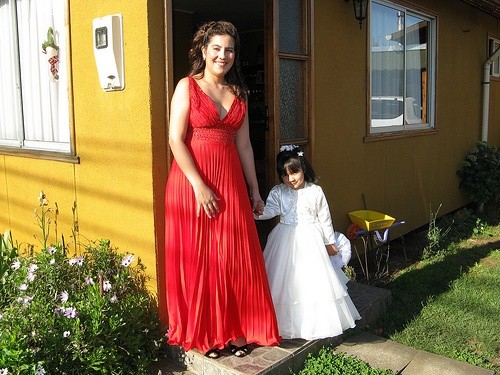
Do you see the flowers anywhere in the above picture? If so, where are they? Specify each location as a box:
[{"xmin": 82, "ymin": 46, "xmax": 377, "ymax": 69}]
[{"xmin": 297, "ymin": 151, "xmax": 304, "ymax": 157}]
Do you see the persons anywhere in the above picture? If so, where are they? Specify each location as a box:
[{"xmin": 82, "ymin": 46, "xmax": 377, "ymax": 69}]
[
  {"xmin": 163, "ymin": 21, "xmax": 282, "ymax": 357},
  {"xmin": 253, "ymin": 144, "xmax": 361, "ymax": 340}
]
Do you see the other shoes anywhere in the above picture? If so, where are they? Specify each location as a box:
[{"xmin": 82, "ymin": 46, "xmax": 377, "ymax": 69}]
[
  {"xmin": 205, "ymin": 349, "xmax": 221, "ymax": 359},
  {"xmin": 230, "ymin": 344, "xmax": 247, "ymax": 357}
]
